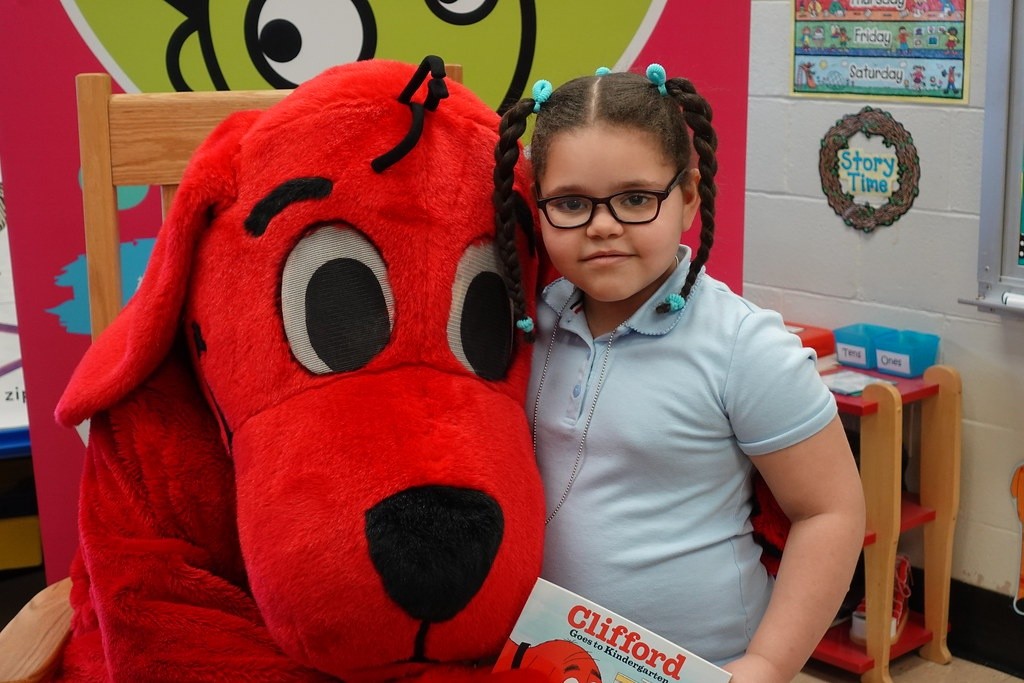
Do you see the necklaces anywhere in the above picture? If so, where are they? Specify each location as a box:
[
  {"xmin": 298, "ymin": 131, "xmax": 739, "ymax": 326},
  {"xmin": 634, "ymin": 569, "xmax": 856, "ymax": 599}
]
[{"xmin": 533, "ymin": 256, "xmax": 679, "ymax": 524}]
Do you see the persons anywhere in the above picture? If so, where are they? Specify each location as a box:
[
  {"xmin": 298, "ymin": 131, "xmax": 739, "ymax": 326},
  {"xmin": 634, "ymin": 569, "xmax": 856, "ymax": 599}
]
[{"xmin": 492, "ymin": 63, "xmax": 867, "ymax": 683}]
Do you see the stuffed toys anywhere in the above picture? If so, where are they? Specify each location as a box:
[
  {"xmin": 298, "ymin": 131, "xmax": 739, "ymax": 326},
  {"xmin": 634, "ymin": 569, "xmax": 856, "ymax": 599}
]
[{"xmin": 0, "ymin": 55, "xmax": 553, "ymax": 683}]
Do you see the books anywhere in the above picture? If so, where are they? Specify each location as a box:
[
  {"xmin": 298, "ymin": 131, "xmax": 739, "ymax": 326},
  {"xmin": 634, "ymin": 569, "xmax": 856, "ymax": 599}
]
[{"xmin": 493, "ymin": 577, "xmax": 732, "ymax": 683}]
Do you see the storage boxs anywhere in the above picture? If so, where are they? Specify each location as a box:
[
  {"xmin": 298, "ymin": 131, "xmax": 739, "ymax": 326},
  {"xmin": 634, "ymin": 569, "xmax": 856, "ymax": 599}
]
[
  {"xmin": 783, "ymin": 322, "xmax": 836, "ymax": 358},
  {"xmin": 873, "ymin": 330, "xmax": 941, "ymax": 379},
  {"xmin": 834, "ymin": 324, "xmax": 898, "ymax": 370}
]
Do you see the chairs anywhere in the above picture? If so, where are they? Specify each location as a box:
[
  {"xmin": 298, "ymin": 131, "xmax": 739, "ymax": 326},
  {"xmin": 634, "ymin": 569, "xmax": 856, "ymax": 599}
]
[{"xmin": 74, "ymin": 63, "xmax": 463, "ymax": 348}]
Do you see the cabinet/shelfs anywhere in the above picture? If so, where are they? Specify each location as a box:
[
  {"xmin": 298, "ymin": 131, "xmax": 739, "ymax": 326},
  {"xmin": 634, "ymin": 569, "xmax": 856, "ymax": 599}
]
[{"xmin": 747, "ymin": 353, "xmax": 962, "ymax": 683}]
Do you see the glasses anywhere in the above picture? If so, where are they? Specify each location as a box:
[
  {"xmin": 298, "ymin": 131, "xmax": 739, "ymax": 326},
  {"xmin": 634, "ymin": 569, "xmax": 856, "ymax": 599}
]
[{"xmin": 536, "ymin": 167, "xmax": 688, "ymax": 229}]
